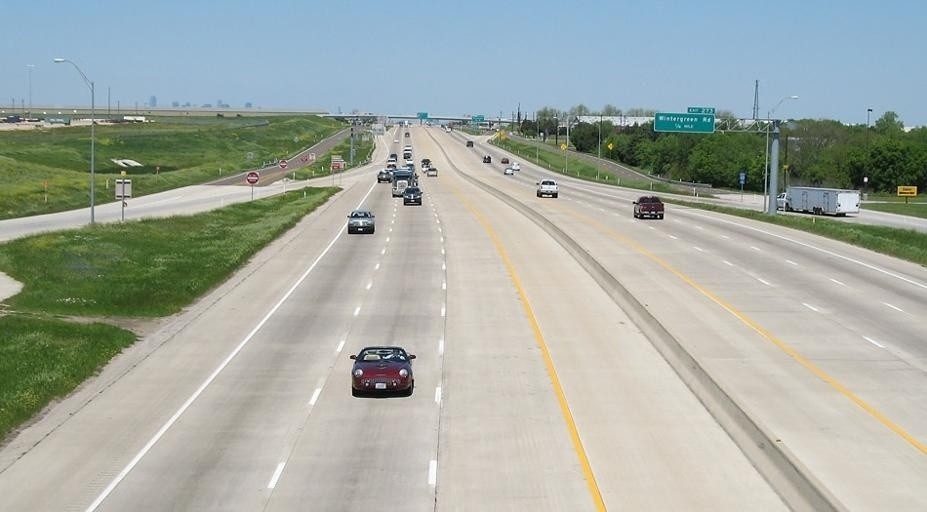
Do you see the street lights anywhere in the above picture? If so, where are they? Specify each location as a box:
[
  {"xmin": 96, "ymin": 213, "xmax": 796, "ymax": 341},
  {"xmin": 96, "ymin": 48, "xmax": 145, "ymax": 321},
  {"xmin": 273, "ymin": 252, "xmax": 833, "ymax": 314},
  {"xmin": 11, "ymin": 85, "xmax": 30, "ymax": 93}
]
[
  {"xmin": 554, "ymin": 117, "xmax": 580, "ymax": 175},
  {"xmin": 763, "ymin": 95, "xmax": 799, "ymax": 214},
  {"xmin": 54, "ymin": 58, "xmax": 93, "ymax": 224}
]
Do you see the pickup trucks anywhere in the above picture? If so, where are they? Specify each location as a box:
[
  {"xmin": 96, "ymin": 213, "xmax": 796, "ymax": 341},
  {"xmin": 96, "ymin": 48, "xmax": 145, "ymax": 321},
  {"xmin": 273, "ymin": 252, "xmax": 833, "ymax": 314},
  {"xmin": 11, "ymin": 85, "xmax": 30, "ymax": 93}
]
[
  {"xmin": 633, "ymin": 196, "xmax": 663, "ymax": 220},
  {"xmin": 536, "ymin": 179, "xmax": 557, "ymax": 198}
]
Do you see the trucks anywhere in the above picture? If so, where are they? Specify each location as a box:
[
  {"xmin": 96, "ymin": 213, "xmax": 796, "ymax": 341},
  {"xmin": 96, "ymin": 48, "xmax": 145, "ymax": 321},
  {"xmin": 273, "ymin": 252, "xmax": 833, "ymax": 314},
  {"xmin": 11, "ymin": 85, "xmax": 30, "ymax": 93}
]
[{"xmin": 778, "ymin": 185, "xmax": 862, "ymax": 216}]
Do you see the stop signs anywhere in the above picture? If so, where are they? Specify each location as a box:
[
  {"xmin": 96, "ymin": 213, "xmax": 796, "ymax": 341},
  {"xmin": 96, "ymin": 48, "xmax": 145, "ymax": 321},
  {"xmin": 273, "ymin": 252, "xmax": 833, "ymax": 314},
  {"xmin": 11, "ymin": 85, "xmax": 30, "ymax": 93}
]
[
  {"xmin": 246, "ymin": 171, "xmax": 259, "ymax": 185},
  {"xmin": 279, "ymin": 160, "xmax": 288, "ymax": 168}
]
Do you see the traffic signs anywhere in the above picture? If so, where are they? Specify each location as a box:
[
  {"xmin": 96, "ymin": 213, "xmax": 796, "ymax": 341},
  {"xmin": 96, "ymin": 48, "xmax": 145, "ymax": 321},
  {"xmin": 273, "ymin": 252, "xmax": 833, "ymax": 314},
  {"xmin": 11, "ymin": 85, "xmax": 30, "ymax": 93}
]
[
  {"xmin": 472, "ymin": 114, "xmax": 484, "ymax": 124},
  {"xmin": 897, "ymin": 186, "xmax": 917, "ymax": 198},
  {"xmin": 654, "ymin": 113, "xmax": 714, "ymax": 134}
]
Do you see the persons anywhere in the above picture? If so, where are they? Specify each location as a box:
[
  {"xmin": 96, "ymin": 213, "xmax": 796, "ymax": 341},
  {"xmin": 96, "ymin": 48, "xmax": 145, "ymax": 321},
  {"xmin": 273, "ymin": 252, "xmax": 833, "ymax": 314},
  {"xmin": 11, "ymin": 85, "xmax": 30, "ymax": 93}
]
[{"xmin": 385, "ymin": 348, "xmax": 405, "ymax": 360}]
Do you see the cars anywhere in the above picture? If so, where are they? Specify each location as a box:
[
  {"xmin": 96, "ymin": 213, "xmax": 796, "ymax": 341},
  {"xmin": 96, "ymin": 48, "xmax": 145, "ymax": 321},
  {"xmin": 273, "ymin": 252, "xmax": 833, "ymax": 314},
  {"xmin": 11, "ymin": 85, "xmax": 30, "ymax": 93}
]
[
  {"xmin": 466, "ymin": 141, "xmax": 473, "ymax": 148},
  {"xmin": 421, "ymin": 159, "xmax": 437, "ymax": 177},
  {"xmin": 348, "ymin": 210, "xmax": 375, "ymax": 234},
  {"xmin": 425, "ymin": 121, "xmax": 452, "ymax": 133},
  {"xmin": 377, "ymin": 145, "xmax": 423, "ymax": 205},
  {"xmin": 502, "ymin": 157, "xmax": 520, "ymax": 175},
  {"xmin": 483, "ymin": 156, "xmax": 491, "ymax": 163},
  {"xmin": 349, "ymin": 346, "xmax": 417, "ymax": 396},
  {"xmin": 394, "ymin": 120, "xmax": 412, "ymax": 143}
]
